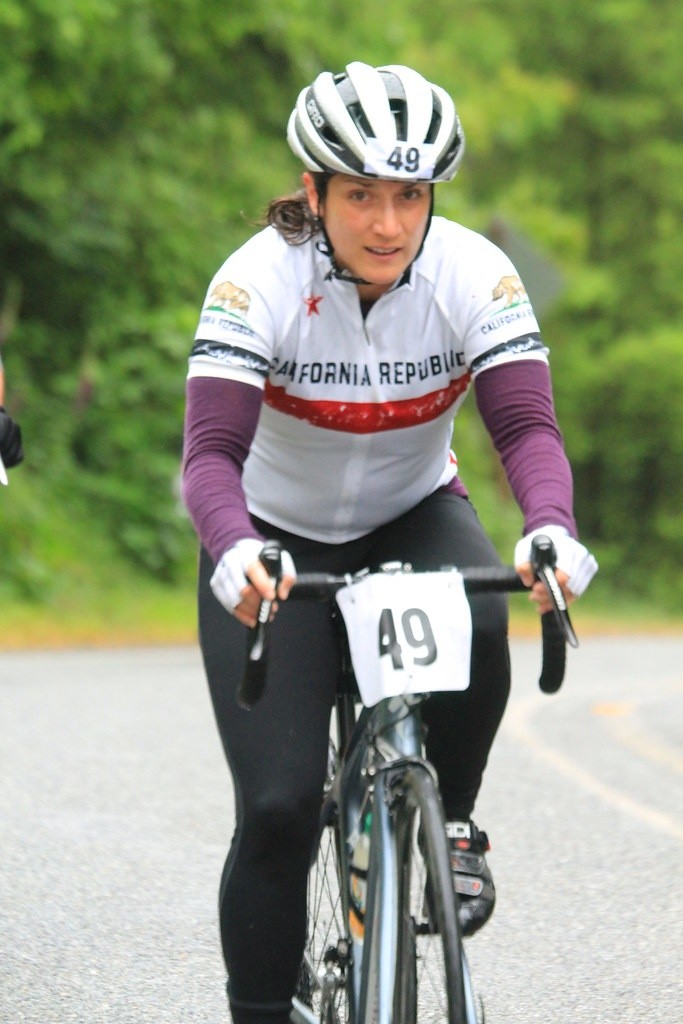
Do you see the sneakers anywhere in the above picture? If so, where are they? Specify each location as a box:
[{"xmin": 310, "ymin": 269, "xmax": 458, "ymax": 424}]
[{"xmin": 416, "ymin": 818, "xmax": 496, "ymax": 936}]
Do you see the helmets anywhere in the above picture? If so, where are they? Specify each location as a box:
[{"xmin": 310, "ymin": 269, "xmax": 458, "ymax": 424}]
[{"xmin": 286, "ymin": 60, "xmax": 465, "ymax": 182}]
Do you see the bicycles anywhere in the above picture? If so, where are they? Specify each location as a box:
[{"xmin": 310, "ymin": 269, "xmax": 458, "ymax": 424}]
[{"xmin": 228, "ymin": 534, "xmax": 583, "ymax": 1024}]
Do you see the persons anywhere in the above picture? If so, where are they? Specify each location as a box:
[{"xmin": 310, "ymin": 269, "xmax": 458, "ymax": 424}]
[{"xmin": 179, "ymin": 61, "xmax": 601, "ymax": 1024}]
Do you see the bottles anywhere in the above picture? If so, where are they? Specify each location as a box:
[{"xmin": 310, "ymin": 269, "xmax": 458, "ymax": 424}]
[{"xmin": 349, "ymin": 813, "xmax": 373, "ymax": 946}]
